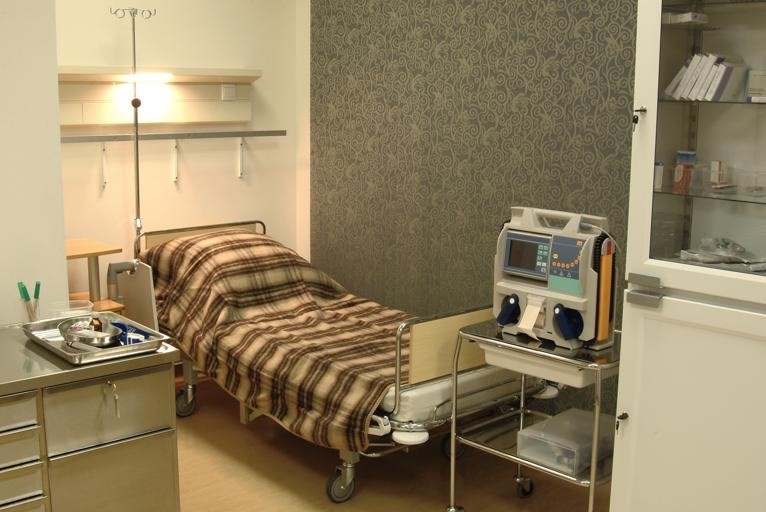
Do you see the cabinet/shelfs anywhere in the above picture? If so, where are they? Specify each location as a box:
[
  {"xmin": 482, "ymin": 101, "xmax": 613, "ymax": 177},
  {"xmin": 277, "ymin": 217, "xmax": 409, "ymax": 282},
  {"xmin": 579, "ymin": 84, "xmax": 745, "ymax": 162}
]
[
  {"xmin": 606, "ymin": 0, "xmax": 766, "ymax": 512},
  {"xmin": 1, "ymin": 364, "xmax": 181, "ymax": 512},
  {"xmin": 448, "ymin": 318, "xmax": 622, "ymax": 512}
]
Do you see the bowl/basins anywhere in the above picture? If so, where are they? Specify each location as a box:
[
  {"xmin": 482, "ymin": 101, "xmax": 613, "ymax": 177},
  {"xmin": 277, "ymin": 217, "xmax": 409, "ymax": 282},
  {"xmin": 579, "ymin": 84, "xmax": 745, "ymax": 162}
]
[{"xmin": 57, "ymin": 316, "xmax": 123, "ymax": 347}]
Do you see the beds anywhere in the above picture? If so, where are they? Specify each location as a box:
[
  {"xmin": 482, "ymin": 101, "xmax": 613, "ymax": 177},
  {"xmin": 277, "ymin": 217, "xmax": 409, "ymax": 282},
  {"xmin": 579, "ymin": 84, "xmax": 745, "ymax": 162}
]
[{"xmin": 134, "ymin": 220, "xmax": 547, "ymax": 505}]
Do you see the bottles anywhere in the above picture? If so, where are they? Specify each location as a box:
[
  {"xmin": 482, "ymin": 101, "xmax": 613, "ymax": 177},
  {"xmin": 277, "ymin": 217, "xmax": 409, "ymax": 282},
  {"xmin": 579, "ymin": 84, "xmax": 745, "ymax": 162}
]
[
  {"xmin": 673, "ymin": 150, "xmax": 700, "ymax": 195},
  {"xmin": 89, "ymin": 311, "xmax": 102, "ymax": 332}
]
